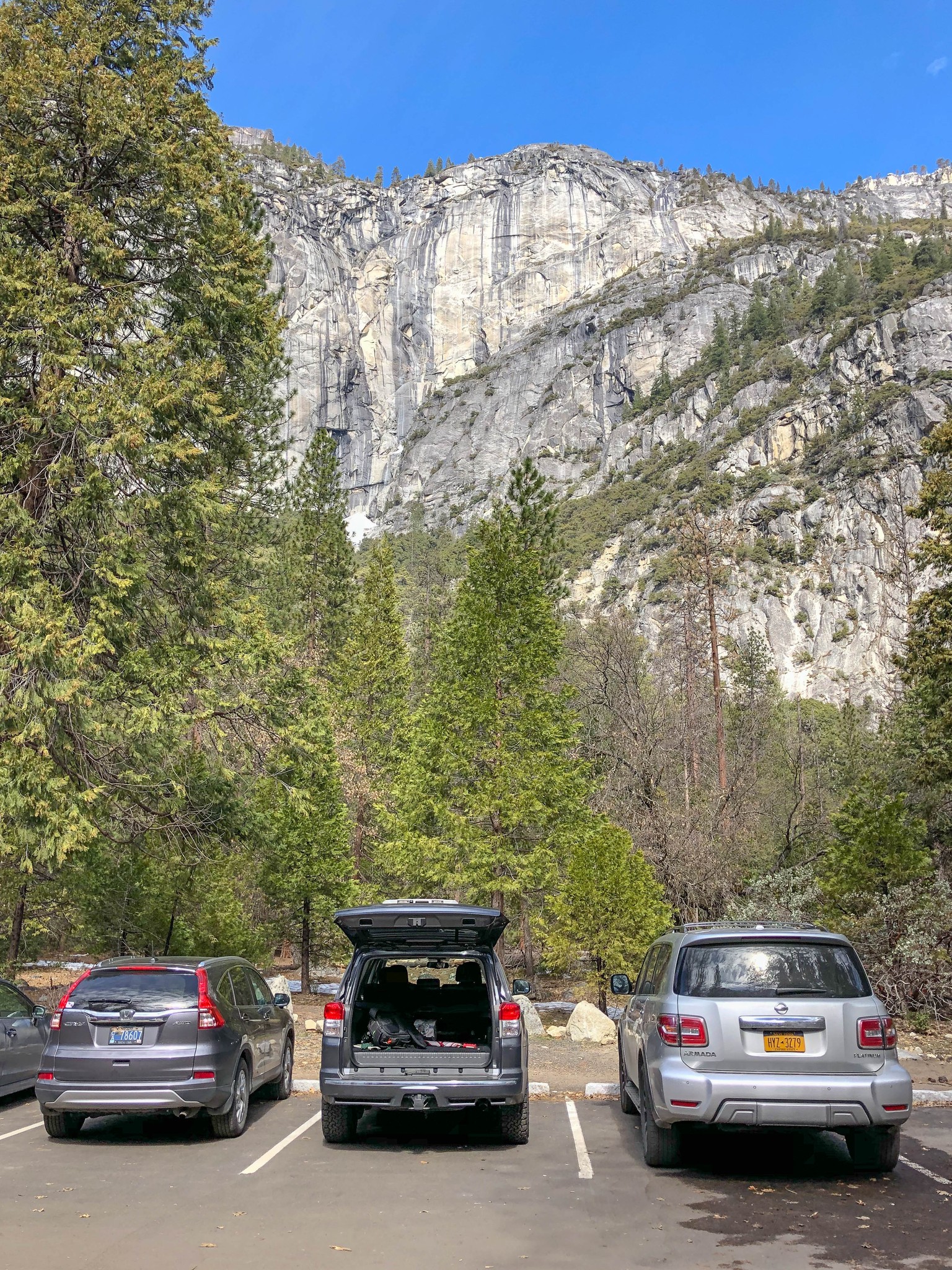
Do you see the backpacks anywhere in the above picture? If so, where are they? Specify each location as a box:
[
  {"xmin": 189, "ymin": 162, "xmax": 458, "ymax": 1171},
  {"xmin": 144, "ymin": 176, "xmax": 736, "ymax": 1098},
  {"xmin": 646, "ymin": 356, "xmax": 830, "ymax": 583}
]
[{"xmin": 368, "ymin": 1015, "xmax": 428, "ymax": 1049}]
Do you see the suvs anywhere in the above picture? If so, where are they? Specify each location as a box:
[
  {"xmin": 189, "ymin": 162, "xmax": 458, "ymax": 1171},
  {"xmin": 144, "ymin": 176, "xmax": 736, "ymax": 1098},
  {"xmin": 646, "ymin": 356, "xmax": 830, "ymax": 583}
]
[
  {"xmin": 318, "ymin": 898, "xmax": 531, "ymax": 1145},
  {"xmin": 34, "ymin": 954, "xmax": 296, "ymax": 1139},
  {"xmin": 610, "ymin": 919, "xmax": 914, "ymax": 1174}
]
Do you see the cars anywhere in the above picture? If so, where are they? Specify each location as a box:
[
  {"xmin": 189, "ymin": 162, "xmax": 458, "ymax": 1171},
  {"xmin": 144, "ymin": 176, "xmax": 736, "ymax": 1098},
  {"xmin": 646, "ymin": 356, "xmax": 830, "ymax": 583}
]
[{"xmin": 0, "ymin": 979, "xmax": 53, "ymax": 1108}]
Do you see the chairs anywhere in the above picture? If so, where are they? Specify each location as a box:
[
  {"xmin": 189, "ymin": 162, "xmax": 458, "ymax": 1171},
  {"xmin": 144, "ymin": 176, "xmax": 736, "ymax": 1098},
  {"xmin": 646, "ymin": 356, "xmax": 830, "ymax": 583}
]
[
  {"xmin": 441, "ymin": 961, "xmax": 492, "ymax": 1028},
  {"xmin": 362, "ymin": 965, "xmax": 414, "ymax": 1027}
]
